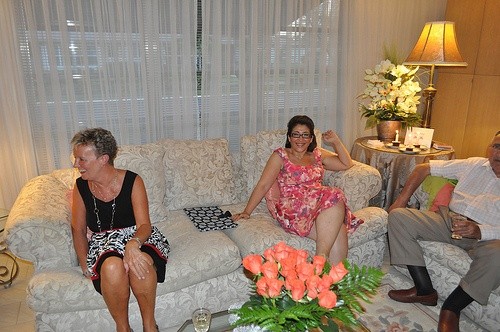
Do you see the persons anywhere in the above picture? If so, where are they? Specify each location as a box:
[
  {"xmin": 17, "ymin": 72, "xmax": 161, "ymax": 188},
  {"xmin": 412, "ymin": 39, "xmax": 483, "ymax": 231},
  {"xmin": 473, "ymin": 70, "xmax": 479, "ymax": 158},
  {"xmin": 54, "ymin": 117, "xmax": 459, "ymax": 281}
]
[
  {"xmin": 231, "ymin": 115, "xmax": 364, "ymax": 266},
  {"xmin": 386, "ymin": 131, "xmax": 500, "ymax": 332},
  {"xmin": 71, "ymin": 128, "xmax": 171, "ymax": 332}
]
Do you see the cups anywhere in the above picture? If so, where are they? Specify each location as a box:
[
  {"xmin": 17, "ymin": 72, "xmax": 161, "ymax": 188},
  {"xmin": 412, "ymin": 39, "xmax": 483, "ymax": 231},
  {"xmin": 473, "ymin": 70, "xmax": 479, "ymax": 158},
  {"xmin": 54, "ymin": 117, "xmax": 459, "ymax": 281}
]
[
  {"xmin": 192, "ymin": 309, "xmax": 211, "ymax": 332},
  {"xmin": 450, "ymin": 215, "xmax": 468, "ymax": 239},
  {"xmin": 392, "ymin": 141, "xmax": 401, "ymax": 146}
]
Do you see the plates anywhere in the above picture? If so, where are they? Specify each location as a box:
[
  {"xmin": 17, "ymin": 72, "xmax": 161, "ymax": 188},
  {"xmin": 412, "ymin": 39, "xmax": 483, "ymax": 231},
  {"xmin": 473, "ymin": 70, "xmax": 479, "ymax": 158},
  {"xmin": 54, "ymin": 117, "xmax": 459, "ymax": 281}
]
[{"xmin": 400, "ymin": 148, "xmax": 421, "ymax": 154}]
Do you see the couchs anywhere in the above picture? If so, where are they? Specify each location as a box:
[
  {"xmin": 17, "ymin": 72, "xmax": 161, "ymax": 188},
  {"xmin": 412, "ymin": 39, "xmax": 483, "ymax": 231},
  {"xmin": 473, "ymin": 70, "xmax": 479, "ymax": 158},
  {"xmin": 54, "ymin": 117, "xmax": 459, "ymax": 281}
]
[
  {"xmin": 392, "ymin": 175, "xmax": 500, "ymax": 332},
  {"xmin": 2, "ymin": 152, "xmax": 388, "ymax": 332}
]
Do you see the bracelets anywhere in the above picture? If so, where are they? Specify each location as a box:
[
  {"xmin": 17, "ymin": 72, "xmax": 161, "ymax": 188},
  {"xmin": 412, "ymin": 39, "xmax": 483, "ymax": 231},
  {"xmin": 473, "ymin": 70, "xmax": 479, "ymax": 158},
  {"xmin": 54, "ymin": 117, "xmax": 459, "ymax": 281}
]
[{"xmin": 130, "ymin": 238, "xmax": 141, "ymax": 246}]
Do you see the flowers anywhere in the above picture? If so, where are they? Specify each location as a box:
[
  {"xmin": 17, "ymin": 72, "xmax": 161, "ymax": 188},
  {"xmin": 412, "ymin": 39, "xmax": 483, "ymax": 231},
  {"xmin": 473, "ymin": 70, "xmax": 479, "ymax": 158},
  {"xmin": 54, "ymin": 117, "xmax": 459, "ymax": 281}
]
[
  {"xmin": 227, "ymin": 242, "xmax": 383, "ymax": 332},
  {"xmin": 352, "ymin": 41, "xmax": 424, "ymax": 132}
]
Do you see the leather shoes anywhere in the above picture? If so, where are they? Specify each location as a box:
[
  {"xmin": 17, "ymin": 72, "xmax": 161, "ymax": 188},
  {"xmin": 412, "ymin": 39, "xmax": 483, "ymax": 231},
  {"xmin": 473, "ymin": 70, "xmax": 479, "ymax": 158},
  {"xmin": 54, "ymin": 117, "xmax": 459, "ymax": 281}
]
[
  {"xmin": 438, "ymin": 310, "xmax": 460, "ymax": 332},
  {"xmin": 388, "ymin": 287, "xmax": 438, "ymax": 307}
]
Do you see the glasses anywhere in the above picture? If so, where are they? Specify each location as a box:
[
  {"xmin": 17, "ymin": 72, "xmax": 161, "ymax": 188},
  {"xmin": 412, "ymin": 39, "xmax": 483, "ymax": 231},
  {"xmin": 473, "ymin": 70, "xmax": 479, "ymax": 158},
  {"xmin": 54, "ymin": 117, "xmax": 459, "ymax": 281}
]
[{"xmin": 291, "ymin": 132, "xmax": 312, "ymax": 139}]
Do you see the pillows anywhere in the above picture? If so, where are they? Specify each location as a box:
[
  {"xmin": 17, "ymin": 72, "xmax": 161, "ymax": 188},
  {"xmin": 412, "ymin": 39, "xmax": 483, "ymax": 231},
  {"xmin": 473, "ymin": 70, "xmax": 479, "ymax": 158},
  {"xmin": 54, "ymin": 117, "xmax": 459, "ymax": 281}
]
[{"xmin": 70, "ymin": 127, "xmax": 322, "ymax": 225}]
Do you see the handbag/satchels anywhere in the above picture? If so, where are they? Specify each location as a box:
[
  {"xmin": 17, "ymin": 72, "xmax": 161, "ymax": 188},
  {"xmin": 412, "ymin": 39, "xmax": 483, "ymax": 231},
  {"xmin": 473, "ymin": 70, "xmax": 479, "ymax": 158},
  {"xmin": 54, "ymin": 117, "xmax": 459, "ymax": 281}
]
[{"xmin": 183, "ymin": 206, "xmax": 238, "ymax": 232}]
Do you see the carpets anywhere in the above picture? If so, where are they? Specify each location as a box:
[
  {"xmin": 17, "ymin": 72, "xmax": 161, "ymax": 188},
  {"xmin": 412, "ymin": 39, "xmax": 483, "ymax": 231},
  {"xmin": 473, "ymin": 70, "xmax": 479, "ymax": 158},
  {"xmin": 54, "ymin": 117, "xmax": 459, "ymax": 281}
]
[{"xmin": 209, "ymin": 280, "xmax": 439, "ymax": 332}]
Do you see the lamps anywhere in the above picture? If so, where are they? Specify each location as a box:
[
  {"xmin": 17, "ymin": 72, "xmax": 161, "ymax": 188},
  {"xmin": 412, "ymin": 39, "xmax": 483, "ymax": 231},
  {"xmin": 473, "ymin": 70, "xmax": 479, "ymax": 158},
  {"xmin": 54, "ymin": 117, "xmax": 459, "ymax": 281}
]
[{"xmin": 402, "ymin": 19, "xmax": 468, "ymax": 128}]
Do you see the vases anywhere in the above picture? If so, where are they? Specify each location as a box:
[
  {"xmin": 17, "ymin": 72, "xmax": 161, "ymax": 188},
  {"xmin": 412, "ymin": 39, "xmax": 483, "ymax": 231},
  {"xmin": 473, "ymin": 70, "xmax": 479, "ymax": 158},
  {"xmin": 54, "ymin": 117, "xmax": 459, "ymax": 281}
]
[{"xmin": 377, "ymin": 120, "xmax": 401, "ymax": 143}]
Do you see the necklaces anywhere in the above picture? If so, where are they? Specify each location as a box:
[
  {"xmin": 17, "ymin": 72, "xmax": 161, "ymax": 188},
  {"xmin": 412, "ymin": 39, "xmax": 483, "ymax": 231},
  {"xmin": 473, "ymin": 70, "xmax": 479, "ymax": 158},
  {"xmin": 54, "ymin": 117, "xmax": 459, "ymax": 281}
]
[{"xmin": 94, "ymin": 195, "xmax": 116, "ymax": 231}]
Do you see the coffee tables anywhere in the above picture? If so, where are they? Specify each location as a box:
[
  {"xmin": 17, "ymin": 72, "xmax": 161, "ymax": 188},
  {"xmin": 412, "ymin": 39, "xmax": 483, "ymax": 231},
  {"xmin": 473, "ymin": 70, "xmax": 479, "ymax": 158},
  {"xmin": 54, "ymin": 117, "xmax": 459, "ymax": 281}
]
[
  {"xmin": 177, "ymin": 308, "xmax": 372, "ymax": 332},
  {"xmin": 349, "ymin": 135, "xmax": 455, "ymax": 211}
]
